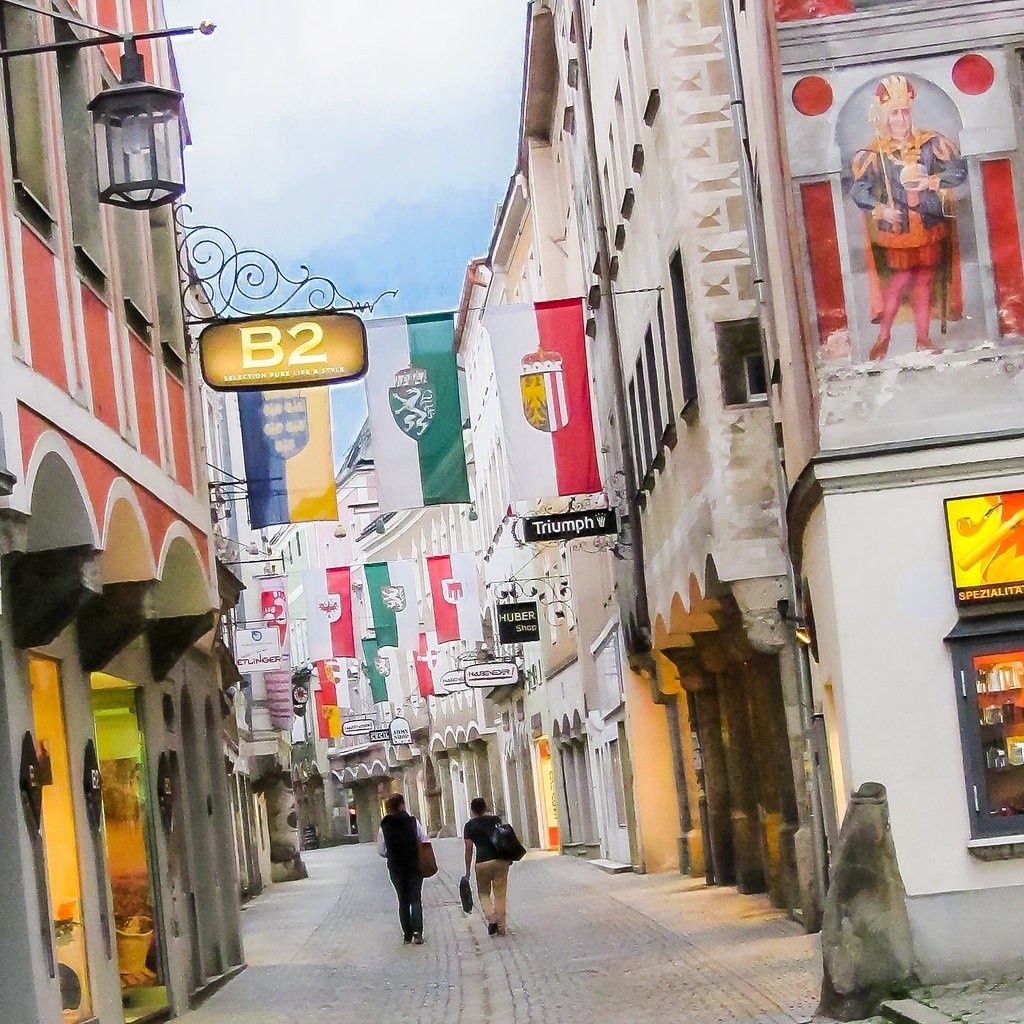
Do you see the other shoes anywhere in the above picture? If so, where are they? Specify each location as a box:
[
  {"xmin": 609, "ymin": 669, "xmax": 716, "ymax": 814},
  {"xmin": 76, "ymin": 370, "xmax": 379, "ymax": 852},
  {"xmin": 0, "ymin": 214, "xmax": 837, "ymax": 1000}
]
[
  {"xmin": 403, "ymin": 934, "xmax": 411, "ymax": 944},
  {"xmin": 487, "ymin": 923, "xmax": 498, "ymax": 935},
  {"xmin": 413, "ymin": 931, "xmax": 422, "ymax": 946}
]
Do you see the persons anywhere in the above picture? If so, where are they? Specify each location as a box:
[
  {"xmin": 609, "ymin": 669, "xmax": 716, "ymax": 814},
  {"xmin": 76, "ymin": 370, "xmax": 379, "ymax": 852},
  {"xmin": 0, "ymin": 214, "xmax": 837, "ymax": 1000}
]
[
  {"xmin": 464, "ymin": 798, "xmax": 512, "ymax": 936},
  {"xmin": 377, "ymin": 793, "xmax": 424, "ymax": 945}
]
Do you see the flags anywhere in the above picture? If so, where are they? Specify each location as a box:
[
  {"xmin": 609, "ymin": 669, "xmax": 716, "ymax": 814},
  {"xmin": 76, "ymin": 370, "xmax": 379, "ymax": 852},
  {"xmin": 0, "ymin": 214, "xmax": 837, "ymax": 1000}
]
[
  {"xmin": 315, "ymin": 658, "xmax": 351, "ymax": 709},
  {"xmin": 423, "ymin": 552, "xmax": 485, "ymax": 643},
  {"xmin": 259, "ymin": 574, "xmax": 290, "ymax": 662},
  {"xmin": 239, "ymin": 383, "xmax": 338, "ymax": 529},
  {"xmin": 315, "ymin": 690, "xmax": 342, "ymax": 739},
  {"xmin": 413, "ymin": 647, "xmax": 454, "ymax": 695},
  {"xmin": 302, "ymin": 566, "xmax": 355, "ymax": 664},
  {"xmin": 484, "ymin": 297, "xmax": 603, "ymax": 498},
  {"xmin": 357, "ymin": 640, "xmax": 401, "ymax": 708},
  {"xmin": 363, "ymin": 558, "xmax": 417, "ymax": 650},
  {"xmin": 362, "ymin": 310, "xmax": 473, "ymax": 511}
]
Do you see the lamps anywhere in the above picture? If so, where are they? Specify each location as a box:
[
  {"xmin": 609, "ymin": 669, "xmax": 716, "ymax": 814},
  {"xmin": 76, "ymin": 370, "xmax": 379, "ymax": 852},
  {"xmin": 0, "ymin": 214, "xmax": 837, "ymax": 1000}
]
[{"xmin": 0, "ymin": 0, "xmax": 217, "ymax": 210}]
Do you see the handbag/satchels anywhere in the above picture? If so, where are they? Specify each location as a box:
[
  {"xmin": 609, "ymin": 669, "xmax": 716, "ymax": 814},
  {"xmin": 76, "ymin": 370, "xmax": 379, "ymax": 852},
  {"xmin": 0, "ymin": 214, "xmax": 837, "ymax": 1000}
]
[
  {"xmin": 412, "ymin": 816, "xmax": 438, "ymax": 878},
  {"xmin": 459, "ymin": 874, "xmax": 473, "ymax": 912},
  {"xmin": 489, "ymin": 818, "xmax": 525, "ymax": 862}
]
